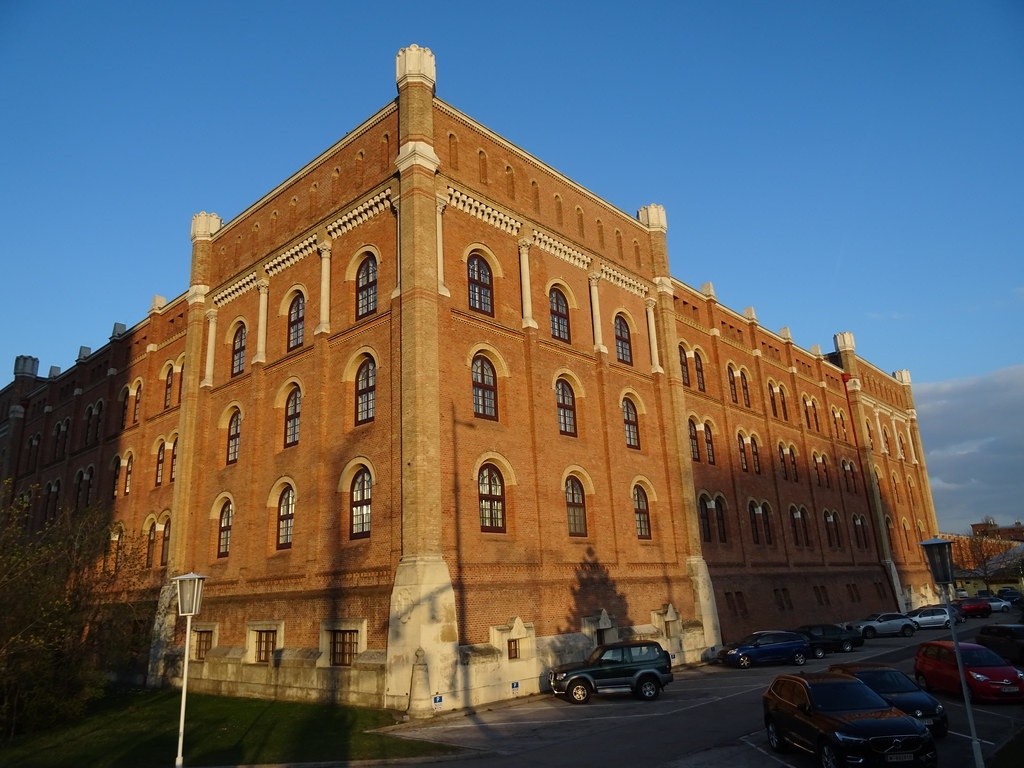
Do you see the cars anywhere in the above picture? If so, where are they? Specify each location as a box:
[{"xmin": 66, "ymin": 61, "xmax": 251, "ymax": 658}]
[
  {"xmin": 904, "ymin": 606, "xmax": 958, "ymax": 631},
  {"xmin": 828, "ymin": 663, "xmax": 948, "ymax": 739},
  {"xmin": 852, "ymin": 612, "xmax": 921, "ymax": 640},
  {"xmin": 723, "ymin": 630, "xmax": 813, "ymax": 669},
  {"xmin": 913, "ymin": 640, "xmax": 1024, "ymax": 706},
  {"xmin": 791, "ymin": 624, "xmax": 865, "ymax": 659},
  {"xmin": 957, "ymin": 588, "xmax": 1024, "ymax": 664}
]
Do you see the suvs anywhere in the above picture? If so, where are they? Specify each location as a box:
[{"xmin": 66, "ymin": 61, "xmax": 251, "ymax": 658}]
[
  {"xmin": 762, "ymin": 669, "xmax": 938, "ymax": 768},
  {"xmin": 547, "ymin": 639, "xmax": 674, "ymax": 705}
]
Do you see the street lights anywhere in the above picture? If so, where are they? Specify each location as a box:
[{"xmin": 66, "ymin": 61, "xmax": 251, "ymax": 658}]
[{"xmin": 169, "ymin": 569, "xmax": 208, "ymax": 768}]
